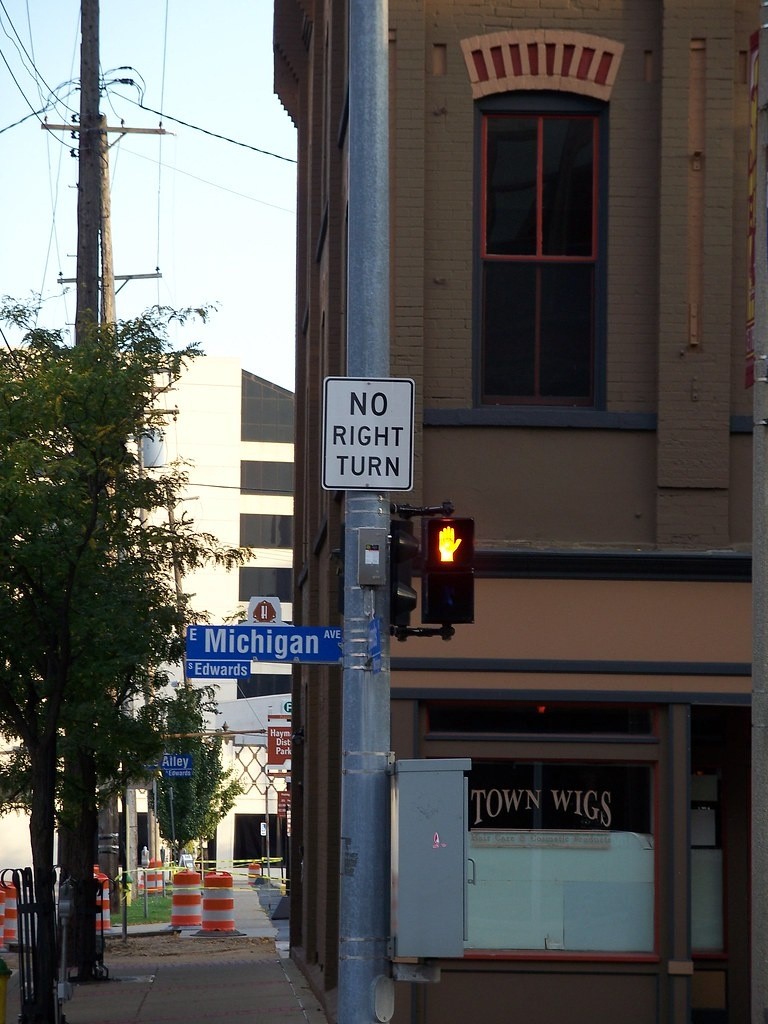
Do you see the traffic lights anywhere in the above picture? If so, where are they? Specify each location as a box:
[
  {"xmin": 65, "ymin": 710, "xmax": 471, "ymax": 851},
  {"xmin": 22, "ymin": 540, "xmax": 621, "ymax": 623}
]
[
  {"xmin": 385, "ymin": 519, "xmax": 421, "ymax": 625},
  {"xmin": 421, "ymin": 517, "xmax": 476, "ymax": 623}
]
